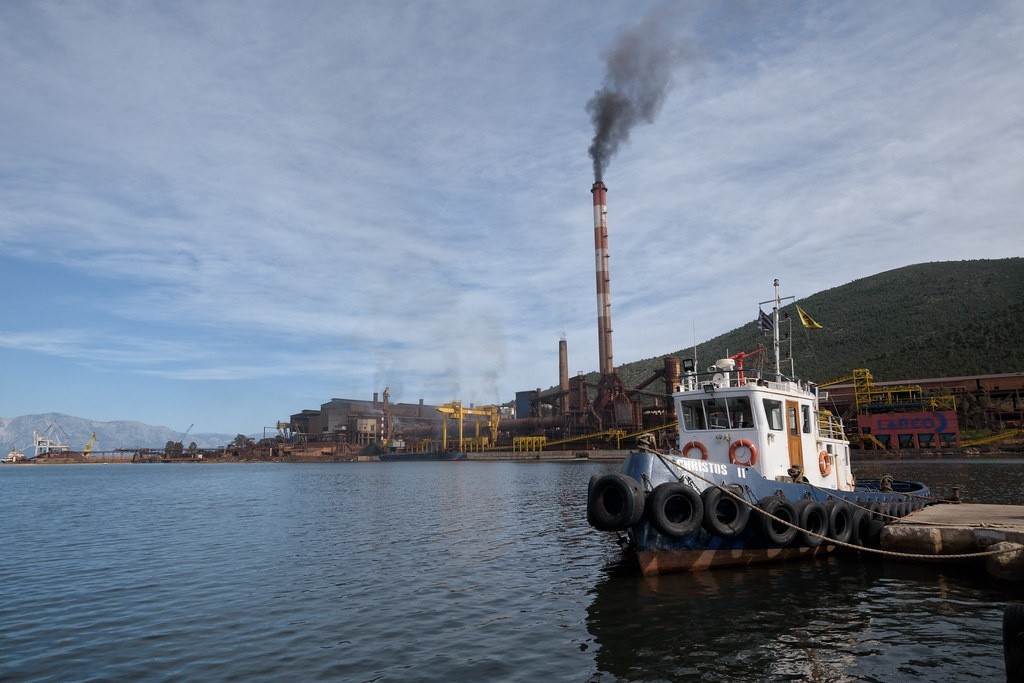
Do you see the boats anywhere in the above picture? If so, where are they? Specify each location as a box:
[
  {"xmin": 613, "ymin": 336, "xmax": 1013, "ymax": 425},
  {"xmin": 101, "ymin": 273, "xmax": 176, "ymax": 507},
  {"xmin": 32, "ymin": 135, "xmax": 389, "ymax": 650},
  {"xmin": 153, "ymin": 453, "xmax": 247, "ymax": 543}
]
[
  {"xmin": 585, "ymin": 278, "xmax": 931, "ymax": 577},
  {"xmin": 1, "ymin": 447, "xmax": 27, "ymax": 463}
]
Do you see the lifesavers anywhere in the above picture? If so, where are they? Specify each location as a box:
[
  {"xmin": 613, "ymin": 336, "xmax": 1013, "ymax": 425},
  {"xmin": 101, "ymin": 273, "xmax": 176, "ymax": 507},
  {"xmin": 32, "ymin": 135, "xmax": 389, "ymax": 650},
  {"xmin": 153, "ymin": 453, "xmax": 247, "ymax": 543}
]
[
  {"xmin": 728, "ymin": 439, "xmax": 758, "ymax": 467},
  {"xmin": 682, "ymin": 441, "xmax": 707, "ymax": 459},
  {"xmin": 819, "ymin": 451, "xmax": 831, "ymax": 477}
]
